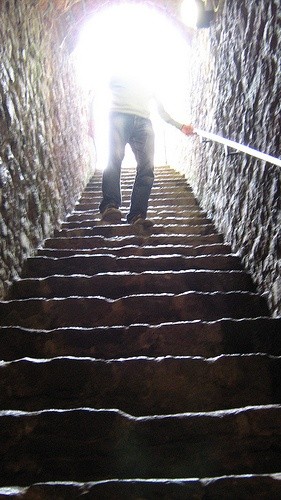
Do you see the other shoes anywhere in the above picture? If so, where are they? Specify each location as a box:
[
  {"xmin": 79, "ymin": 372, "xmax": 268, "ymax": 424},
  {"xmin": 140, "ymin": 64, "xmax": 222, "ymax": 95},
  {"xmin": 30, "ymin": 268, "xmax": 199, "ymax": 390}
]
[
  {"xmin": 101, "ymin": 207, "xmax": 122, "ymax": 221},
  {"xmin": 129, "ymin": 216, "xmax": 155, "ymax": 227}
]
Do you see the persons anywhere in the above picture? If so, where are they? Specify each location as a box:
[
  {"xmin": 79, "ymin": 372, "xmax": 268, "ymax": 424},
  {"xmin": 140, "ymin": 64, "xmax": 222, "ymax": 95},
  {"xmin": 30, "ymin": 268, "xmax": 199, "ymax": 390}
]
[{"xmin": 89, "ymin": 72, "xmax": 195, "ymax": 228}]
